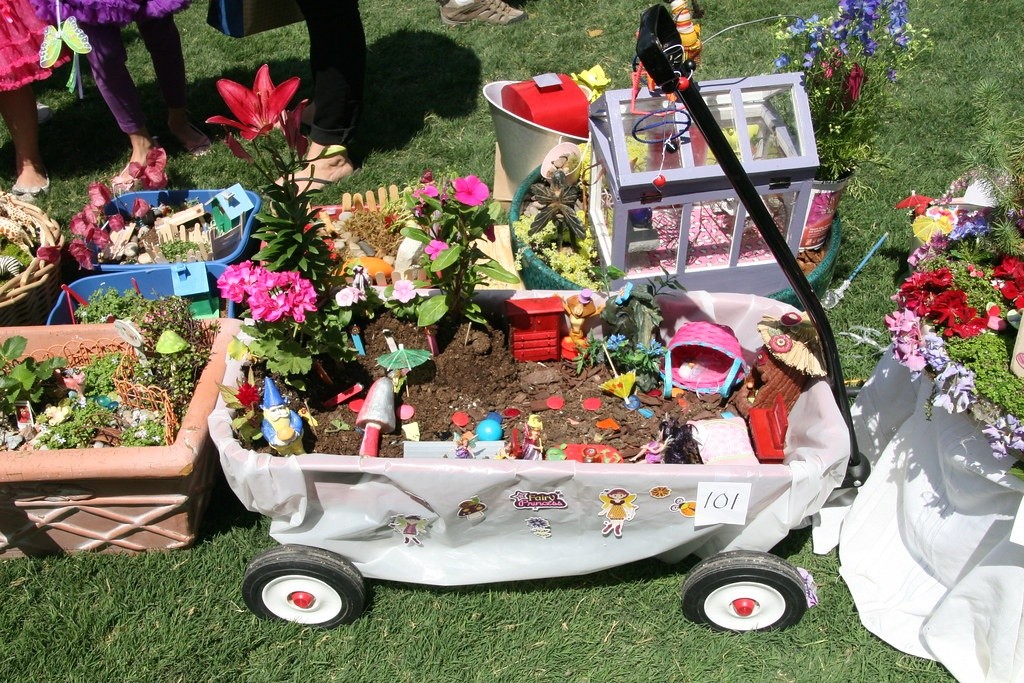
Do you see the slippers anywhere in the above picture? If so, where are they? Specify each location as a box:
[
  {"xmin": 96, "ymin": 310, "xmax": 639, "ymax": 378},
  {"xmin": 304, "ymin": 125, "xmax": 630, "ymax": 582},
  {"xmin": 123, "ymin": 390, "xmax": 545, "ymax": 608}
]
[
  {"xmin": 274, "ymin": 158, "xmax": 360, "ymax": 196},
  {"xmin": 290, "ymin": 122, "xmax": 313, "ymax": 133}
]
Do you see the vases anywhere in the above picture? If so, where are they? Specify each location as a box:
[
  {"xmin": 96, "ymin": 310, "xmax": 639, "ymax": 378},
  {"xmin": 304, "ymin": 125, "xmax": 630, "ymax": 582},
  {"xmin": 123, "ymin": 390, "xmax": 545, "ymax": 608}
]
[
  {"xmin": 0, "ymin": 316, "xmax": 242, "ymax": 559},
  {"xmin": 260, "ymin": 185, "xmax": 450, "ymax": 288},
  {"xmin": 45, "ymin": 262, "xmax": 235, "ymax": 326},
  {"xmin": 796, "ymin": 167, "xmax": 852, "ymax": 253},
  {"xmin": 0, "ymin": 191, "xmax": 64, "ymax": 327},
  {"xmin": 510, "ymin": 134, "xmax": 843, "ymax": 311},
  {"xmin": 86, "ymin": 190, "xmax": 263, "ymax": 273}
]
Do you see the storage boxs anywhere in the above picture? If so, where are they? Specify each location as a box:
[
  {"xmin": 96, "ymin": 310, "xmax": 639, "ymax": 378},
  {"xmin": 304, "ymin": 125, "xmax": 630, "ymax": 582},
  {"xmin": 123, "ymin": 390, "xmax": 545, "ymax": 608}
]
[{"xmin": 586, "ymin": 70, "xmax": 820, "ymax": 298}]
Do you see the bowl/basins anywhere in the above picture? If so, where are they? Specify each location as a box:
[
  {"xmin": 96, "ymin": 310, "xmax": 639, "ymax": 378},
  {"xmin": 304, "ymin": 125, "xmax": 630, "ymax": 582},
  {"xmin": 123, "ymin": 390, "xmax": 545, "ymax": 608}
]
[{"xmin": 483, "ymin": 80, "xmax": 591, "ymax": 183}]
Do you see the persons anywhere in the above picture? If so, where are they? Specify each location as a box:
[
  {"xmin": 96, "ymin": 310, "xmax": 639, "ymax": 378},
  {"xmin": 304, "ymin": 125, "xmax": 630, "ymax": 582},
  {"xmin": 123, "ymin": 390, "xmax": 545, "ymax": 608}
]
[{"xmin": 1, "ymin": 0, "xmax": 531, "ymax": 199}]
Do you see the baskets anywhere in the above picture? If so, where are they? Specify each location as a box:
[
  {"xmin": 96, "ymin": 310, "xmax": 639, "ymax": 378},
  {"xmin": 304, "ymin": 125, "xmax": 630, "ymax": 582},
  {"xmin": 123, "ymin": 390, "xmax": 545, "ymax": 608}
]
[
  {"xmin": 144, "ymin": 224, "xmax": 217, "ymax": 263},
  {"xmin": 1, "ymin": 191, "xmax": 65, "ymax": 327},
  {"xmin": 658, "ymin": 322, "xmax": 748, "ymax": 401},
  {"xmin": 188, "ymin": 207, "xmax": 244, "ymax": 258}
]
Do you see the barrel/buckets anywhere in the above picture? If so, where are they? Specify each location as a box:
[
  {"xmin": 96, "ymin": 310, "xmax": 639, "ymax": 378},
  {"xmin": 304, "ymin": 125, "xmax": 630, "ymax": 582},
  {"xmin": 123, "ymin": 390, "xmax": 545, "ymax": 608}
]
[{"xmin": 500, "ymin": 73, "xmax": 590, "ymax": 138}]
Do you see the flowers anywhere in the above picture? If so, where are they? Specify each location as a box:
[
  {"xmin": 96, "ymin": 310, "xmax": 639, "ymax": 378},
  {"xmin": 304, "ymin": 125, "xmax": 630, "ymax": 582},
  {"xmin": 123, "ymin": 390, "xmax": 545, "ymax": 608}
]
[{"xmin": 0, "ymin": 0, "xmax": 1024, "ymax": 480}]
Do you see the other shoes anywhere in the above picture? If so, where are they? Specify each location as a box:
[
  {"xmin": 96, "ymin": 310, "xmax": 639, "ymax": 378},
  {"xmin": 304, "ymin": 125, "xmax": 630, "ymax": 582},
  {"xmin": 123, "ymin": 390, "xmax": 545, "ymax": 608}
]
[
  {"xmin": 168, "ymin": 119, "xmax": 212, "ymax": 156},
  {"xmin": 112, "ymin": 137, "xmax": 158, "ymax": 193},
  {"xmin": 13, "ymin": 161, "xmax": 50, "ymax": 196}
]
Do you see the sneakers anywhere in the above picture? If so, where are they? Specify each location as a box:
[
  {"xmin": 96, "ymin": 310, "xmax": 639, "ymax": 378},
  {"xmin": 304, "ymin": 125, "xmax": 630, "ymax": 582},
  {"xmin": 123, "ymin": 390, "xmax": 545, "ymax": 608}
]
[{"xmin": 436, "ymin": 0, "xmax": 525, "ymax": 26}]
